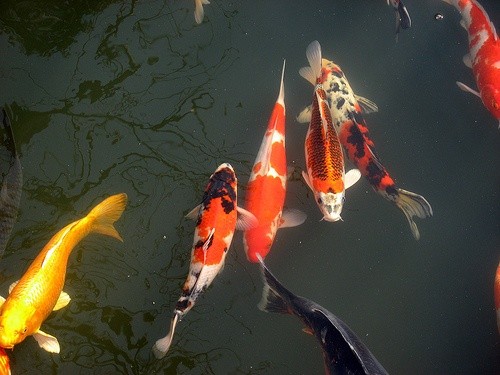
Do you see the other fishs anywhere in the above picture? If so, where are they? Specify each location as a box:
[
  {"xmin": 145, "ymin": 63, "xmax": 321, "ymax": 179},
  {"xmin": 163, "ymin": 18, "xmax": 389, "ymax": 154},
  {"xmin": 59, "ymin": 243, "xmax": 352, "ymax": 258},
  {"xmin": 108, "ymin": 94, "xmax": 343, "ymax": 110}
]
[
  {"xmin": 0, "ymin": 109, "xmax": 23, "ymax": 256},
  {"xmin": 257, "ymin": 253, "xmax": 391, "ymax": 375}
]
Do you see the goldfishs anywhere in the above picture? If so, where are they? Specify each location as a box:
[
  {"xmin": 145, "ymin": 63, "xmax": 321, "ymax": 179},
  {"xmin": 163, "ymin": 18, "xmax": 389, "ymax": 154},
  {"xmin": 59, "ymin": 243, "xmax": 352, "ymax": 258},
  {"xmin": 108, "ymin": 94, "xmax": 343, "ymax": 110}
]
[
  {"xmin": 299, "ymin": 39, "xmax": 361, "ymax": 223},
  {"xmin": 297, "ymin": 58, "xmax": 432, "ymax": 241},
  {"xmin": 444, "ymin": 1, "xmax": 500, "ymax": 123},
  {"xmin": 0, "ymin": 192, "xmax": 128, "ymax": 354},
  {"xmin": 243, "ymin": 58, "xmax": 307, "ymax": 263},
  {"xmin": 153, "ymin": 163, "xmax": 259, "ymax": 361}
]
